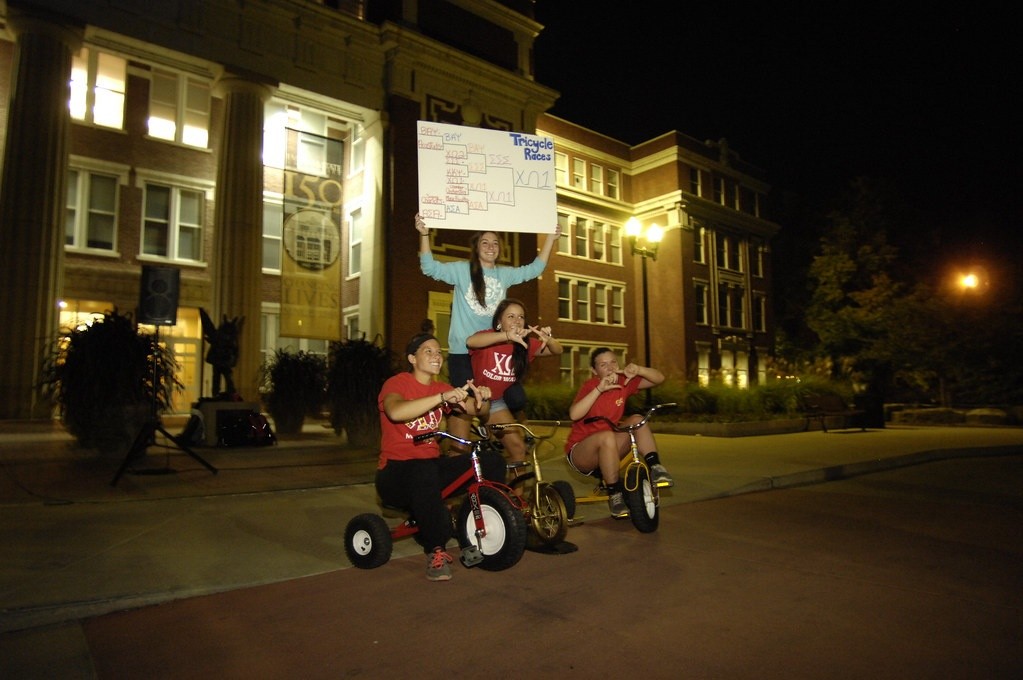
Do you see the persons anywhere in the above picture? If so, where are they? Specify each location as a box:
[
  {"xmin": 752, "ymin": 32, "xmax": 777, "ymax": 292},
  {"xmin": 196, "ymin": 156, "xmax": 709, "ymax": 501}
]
[
  {"xmin": 199, "ymin": 305, "xmax": 244, "ymax": 396},
  {"xmin": 564, "ymin": 347, "xmax": 673, "ymax": 516},
  {"xmin": 378, "ymin": 333, "xmax": 491, "ymax": 580},
  {"xmin": 420, "ymin": 319, "xmax": 435, "ymax": 336},
  {"xmin": 415, "ymin": 212, "xmax": 562, "ymax": 458},
  {"xmin": 466, "ymin": 298, "xmax": 563, "ymax": 506}
]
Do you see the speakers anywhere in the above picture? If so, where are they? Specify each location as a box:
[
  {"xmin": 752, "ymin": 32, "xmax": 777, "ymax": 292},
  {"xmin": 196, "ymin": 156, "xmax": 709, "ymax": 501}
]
[{"xmin": 135, "ymin": 263, "xmax": 182, "ymax": 327}]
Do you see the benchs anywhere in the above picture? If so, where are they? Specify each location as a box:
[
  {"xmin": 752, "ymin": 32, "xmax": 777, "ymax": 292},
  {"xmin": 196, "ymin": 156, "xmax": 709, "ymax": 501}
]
[{"xmin": 797, "ymin": 390, "xmax": 867, "ymax": 431}]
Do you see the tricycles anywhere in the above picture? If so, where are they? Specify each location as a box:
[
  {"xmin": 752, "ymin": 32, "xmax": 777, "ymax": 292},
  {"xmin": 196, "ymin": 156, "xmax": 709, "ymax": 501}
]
[
  {"xmin": 483, "ymin": 421, "xmax": 577, "ymax": 546},
  {"xmin": 573, "ymin": 400, "xmax": 680, "ymax": 533},
  {"xmin": 342, "ymin": 428, "xmax": 528, "ymax": 573}
]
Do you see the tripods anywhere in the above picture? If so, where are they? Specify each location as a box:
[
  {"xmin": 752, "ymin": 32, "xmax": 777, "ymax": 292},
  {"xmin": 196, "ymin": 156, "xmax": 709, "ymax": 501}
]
[{"xmin": 108, "ymin": 326, "xmax": 218, "ymax": 487}]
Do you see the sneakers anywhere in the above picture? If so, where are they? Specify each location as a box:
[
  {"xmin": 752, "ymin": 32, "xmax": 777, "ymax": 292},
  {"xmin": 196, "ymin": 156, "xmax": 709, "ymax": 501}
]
[
  {"xmin": 650, "ymin": 465, "xmax": 672, "ymax": 483},
  {"xmin": 427, "ymin": 548, "xmax": 452, "ymax": 580},
  {"xmin": 609, "ymin": 494, "xmax": 631, "ymax": 514}
]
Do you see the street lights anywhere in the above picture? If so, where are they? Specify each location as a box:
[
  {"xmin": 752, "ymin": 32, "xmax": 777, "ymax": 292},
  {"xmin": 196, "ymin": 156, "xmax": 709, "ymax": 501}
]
[{"xmin": 624, "ymin": 216, "xmax": 666, "ymax": 413}]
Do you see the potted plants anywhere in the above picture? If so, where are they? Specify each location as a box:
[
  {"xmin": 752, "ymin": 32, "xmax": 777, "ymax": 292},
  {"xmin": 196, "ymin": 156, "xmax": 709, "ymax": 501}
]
[
  {"xmin": 39, "ymin": 304, "xmax": 185, "ymax": 452},
  {"xmin": 327, "ymin": 337, "xmax": 401, "ymax": 449},
  {"xmin": 255, "ymin": 346, "xmax": 325, "ymax": 433}
]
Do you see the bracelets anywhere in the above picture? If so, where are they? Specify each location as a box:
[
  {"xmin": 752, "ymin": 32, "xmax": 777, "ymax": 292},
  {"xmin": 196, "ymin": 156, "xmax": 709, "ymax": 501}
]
[
  {"xmin": 596, "ymin": 386, "xmax": 602, "ymax": 393},
  {"xmin": 421, "ymin": 234, "xmax": 429, "ymax": 236},
  {"xmin": 506, "ymin": 331, "xmax": 509, "ymax": 341}
]
